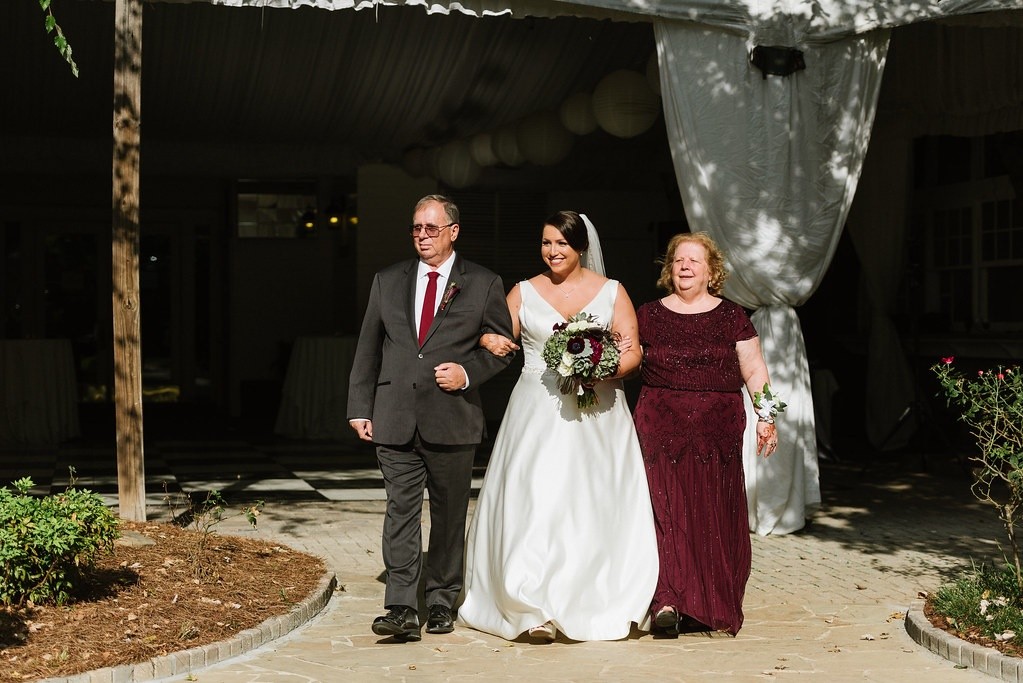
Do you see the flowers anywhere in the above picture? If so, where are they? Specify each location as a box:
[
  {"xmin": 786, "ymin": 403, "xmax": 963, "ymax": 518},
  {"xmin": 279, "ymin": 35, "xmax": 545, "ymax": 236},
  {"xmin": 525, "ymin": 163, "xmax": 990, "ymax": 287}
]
[
  {"xmin": 442, "ymin": 281, "xmax": 461, "ymax": 311},
  {"xmin": 753, "ymin": 382, "xmax": 788, "ymax": 424},
  {"xmin": 541, "ymin": 311, "xmax": 623, "ymax": 411}
]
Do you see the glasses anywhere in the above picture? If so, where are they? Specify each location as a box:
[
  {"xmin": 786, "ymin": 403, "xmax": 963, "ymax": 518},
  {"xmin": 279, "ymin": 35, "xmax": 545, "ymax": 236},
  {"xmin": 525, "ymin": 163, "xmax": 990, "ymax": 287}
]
[{"xmin": 410, "ymin": 222, "xmax": 458, "ymax": 237}]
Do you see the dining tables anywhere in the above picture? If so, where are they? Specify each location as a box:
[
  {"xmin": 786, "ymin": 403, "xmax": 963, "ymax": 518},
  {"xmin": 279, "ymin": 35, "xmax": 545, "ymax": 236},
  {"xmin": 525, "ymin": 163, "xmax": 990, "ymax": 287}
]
[
  {"xmin": 0, "ymin": 337, "xmax": 81, "ymax": 445},
  {"xmin": 276, "ymin": 335, "xmax": 360, "ymax": 441}
]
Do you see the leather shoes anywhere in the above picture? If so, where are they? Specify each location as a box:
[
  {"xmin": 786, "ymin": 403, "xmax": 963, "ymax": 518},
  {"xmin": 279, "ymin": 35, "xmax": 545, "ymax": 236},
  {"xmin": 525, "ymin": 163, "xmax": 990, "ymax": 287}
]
[
  {"xmin": 425, "ymin": 605, "xmax": 454, "ymax": 633},
  {"xmin": 372, "ymin": 605, "xmax": 421, "ymax": 641}
]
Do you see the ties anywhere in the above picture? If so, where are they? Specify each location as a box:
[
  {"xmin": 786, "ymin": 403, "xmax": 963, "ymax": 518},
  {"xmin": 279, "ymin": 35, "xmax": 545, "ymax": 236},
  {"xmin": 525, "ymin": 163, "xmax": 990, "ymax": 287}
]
[{"xmin": 418, "ymin": 272, "xmax": 439, "ymax": 348}]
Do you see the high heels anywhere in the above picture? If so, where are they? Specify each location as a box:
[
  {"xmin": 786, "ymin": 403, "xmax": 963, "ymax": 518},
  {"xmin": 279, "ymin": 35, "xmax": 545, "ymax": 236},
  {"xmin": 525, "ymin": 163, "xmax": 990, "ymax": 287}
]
[
  {"xmin": 528, "ymin": 621, "xmax": 556, "ymax": 642},
  {"xmin": 655, "ymin": 604, "xmax": 680, "ymax": 635}
]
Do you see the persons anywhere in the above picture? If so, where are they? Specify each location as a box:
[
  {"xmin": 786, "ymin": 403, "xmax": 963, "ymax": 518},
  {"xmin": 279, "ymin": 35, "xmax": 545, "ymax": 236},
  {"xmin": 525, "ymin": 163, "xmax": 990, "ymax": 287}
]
[
  {"xmin": 477, "ymin": 209, "xmax": 642, "ymax": 643},
  {"xmin": 619, "ymin": 233, "xmax": 786, "ymax": 641},
  {"xmin": 344, "ymin": 195, "xmax": 518, "ymax": 642}
]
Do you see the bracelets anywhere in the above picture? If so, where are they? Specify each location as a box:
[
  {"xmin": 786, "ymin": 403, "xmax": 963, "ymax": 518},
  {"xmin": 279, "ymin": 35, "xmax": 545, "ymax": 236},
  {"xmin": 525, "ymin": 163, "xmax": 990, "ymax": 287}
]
[{"xmin": 757, "ymin": 419, "xmax": 776, "ymax": 424}]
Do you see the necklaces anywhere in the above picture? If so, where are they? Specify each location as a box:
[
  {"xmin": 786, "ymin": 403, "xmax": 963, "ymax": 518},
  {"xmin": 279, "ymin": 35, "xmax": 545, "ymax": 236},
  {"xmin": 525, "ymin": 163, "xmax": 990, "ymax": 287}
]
[{"xmin": 546, "ymin": 268, "xmax": 587, "ymax": 299}]
